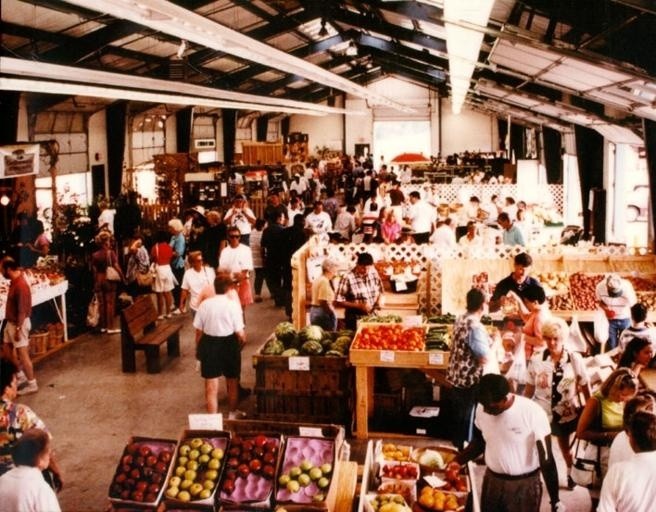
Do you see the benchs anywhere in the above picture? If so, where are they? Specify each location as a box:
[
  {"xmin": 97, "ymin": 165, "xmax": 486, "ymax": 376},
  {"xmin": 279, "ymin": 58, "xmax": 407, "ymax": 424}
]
[{"xmin": 120, "ymin": 295, "xmax": 184, "ymax": 374}]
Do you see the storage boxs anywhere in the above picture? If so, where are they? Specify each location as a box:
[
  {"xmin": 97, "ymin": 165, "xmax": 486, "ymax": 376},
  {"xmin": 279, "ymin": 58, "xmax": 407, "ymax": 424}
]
[
  {"xmin": 379, "ymin": 445, "xmax": 420, "ymax": 502},
  {"xmin": 432, "ymin": 471, "xmax": 471, "ymax": 508},
  {"xmin": 106, "ymin": 428, "xmax": 335, "ymax": 512}
]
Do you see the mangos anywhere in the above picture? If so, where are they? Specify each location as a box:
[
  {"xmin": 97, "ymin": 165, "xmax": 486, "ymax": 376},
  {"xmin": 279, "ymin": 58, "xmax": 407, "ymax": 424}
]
[{"xmin": 382, "ymin": 444, "xmax": 409, "ymax": 461}]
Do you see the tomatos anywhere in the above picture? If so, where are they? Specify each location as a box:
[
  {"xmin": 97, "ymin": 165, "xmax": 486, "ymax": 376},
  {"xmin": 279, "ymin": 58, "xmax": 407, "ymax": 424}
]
[{"xmin": 353, "ymin": 325, "xmax": 424, "ymax": 352}]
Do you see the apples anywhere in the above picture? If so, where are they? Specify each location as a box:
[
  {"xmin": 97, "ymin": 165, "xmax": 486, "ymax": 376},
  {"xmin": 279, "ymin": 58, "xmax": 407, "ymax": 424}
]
[
  {"xmin": 30, "ymin": 323, "xmax": 64, "ymax": 335},
  {"xmin": 442, "ymin": 462, "xmax": 465, "ymax": 491},
  {"xmin": 384, "ymin": 462, "xmax": 417, "ymax": 481},
  {"xmin": 110, "ymin": 434, "xmax": 279, "ymax": 502},
  {"xmin": 280, "ymin": 461, "xmax": 333, "ymax": 503}
]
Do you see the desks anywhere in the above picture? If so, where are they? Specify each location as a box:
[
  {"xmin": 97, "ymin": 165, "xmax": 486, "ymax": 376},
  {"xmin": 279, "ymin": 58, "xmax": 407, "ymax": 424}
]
[
  {"xmin": 352, "ymin": 362, "xmax": 448, "ymax": 442},
  {"xmin": 0, "ymin": 280, "xmax": 69, "ymax": 343}
]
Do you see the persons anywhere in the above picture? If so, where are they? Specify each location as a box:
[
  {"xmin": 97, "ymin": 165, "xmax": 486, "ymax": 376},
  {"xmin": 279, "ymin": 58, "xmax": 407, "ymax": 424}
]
[{"xmin": 0, "ymin": 143, "xmax": 656, "ymax": 511}]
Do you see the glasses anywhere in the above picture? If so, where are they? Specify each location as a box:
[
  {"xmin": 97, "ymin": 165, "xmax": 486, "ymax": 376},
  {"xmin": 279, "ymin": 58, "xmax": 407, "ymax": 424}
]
[{"xmin": 229, "ymin": 235, "xmax": 238, "ymax": 239}]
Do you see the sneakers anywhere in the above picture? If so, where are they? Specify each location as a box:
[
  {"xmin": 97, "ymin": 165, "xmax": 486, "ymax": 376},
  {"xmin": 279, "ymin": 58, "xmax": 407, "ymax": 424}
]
[
  {"xmin": 17, "ymin": 380, "xmax": 38, "ymax": 395},
  {"xmin": 16, "ymin": 373, "xmax": 27, "ymax": 386},
  {"xmin": 158, "ymin": 304, "xmax": 186, "ymax": 320}
]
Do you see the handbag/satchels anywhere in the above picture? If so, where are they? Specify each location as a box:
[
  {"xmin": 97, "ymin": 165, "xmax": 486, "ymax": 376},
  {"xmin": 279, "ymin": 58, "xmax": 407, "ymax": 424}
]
[
  {"xmin": 106, "ymin": 251, "xmax": 121, "ymax": 281},
  {"xmin": 569, "ymin": 397, "xmax": 600, "ymax": 489},
  {"xmin": 85, "ymin": 294, "xmax": 100, "ymax": 326},
  {"xmin": 136, "ymin": 270, "xmax": 154, "ymax": 287}
]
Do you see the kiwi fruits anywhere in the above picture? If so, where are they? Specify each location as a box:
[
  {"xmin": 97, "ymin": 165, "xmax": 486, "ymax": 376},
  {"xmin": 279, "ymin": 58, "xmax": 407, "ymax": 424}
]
[{"xmin": 370, "ymin": 495, "xmax": 405, "ymax": 510}]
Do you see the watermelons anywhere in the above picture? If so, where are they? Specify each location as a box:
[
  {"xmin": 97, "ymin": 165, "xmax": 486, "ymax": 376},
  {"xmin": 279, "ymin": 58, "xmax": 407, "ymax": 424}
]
[{"xmin": 263, "ymin": 322, "xmax": 353, "ymax": 356}]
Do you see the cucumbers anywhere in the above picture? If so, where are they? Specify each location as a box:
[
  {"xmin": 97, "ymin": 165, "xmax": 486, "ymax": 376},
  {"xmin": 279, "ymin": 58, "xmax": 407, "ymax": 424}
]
[{"xmin": 425, "ymin": 313, "xmax": 456, "ymax": 351}]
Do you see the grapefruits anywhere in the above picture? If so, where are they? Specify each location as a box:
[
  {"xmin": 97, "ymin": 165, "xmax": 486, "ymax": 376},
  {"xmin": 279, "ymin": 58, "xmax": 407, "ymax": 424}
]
[
  {"xmin": 379, "ymin": 503, "xmax": 405, "ymax": 512},
  {"xmin": 419, "ymin": 449, "xmax": 443, "ymax": 469}
]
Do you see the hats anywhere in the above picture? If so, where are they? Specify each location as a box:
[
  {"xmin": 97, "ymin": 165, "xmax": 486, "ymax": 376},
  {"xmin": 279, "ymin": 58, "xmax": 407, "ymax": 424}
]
[
  {"xmin": 191, "ymin": 204, "xmax": 207, "ymax": 218},
  {"xmin": 606, "ymin": 275, "xmax": 623, "ymax": 298}
]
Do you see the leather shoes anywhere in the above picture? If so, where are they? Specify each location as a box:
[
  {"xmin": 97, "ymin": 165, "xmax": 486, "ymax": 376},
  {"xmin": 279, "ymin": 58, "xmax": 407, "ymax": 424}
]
[
  {"xmin": 100, "ymin": 327, "xmax": 107, "ymax": 334},
  {"xmin": 108, "ymin": 329, "xmax": 122, "ymax": 334}
]
